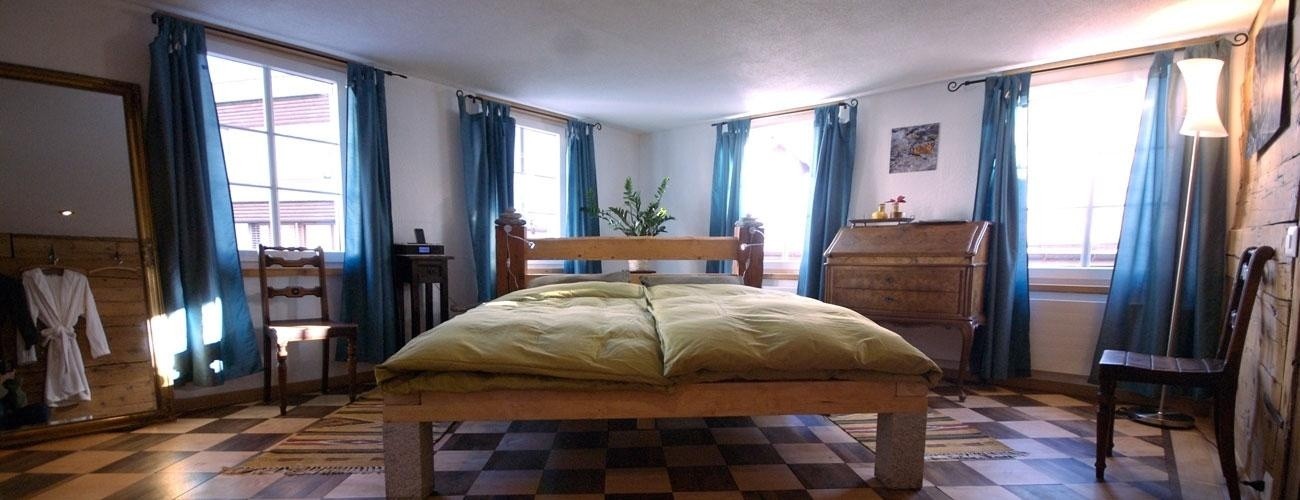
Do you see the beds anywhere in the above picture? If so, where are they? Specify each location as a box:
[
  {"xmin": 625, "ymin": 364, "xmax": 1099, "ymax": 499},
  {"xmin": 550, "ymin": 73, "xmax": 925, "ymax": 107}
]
[{"xmin": 373, "ymin": 207, "xmax": 936, "ymax": 500}]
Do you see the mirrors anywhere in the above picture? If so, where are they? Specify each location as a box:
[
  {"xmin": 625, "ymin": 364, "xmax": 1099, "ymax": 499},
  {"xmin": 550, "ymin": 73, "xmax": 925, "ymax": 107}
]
[{"xmin": 0, "ymin": 63, "xmax": 181, "ymax": 448}]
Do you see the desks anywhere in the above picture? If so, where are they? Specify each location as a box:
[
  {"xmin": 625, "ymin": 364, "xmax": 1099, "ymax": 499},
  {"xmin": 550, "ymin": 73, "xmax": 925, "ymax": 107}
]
[{"xmin": 391, "ymin": 252, "xmax": 455, "ymax": 352}]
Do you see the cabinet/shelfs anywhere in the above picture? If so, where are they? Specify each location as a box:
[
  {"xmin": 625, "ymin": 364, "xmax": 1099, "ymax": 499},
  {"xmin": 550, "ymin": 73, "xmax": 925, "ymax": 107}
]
[{"xmin": 821, "ymin": 221, "xmax": 990, "ymax": 386}]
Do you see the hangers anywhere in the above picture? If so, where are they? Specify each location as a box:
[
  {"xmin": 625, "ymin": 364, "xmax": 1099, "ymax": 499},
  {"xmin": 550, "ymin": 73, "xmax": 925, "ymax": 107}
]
[{"xmin": 23, "ymin": 253, "xmax": 140, "ymax": 276}]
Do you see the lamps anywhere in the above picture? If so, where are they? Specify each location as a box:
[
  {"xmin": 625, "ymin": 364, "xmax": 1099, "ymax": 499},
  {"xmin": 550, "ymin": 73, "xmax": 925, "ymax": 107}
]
[{"xmin": 1129, "ymin": 54, "xmax": 1234, "ymax": 433}]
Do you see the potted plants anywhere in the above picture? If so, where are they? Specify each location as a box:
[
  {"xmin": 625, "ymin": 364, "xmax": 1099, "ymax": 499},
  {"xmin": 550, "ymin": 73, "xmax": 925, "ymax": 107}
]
[{"xmin": 580, "ymin": 173, "xmax": 679, "ymax": 274}]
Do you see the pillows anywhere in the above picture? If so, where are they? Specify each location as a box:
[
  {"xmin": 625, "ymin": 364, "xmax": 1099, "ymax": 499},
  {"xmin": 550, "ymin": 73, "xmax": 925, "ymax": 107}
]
[{"xmin": 524, "ymin": 270, "xmax": 745, "ymax": 289}]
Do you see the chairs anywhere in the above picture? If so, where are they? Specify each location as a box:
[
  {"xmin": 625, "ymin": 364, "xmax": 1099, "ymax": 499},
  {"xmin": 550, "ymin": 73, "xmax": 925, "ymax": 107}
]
[
  {"xmin": 256, "ymin": 243, "xmax": 360, "ymax": 417},
  {"xmin": 1093, "ymin": 245, "xmax": 1277, "ymax": 500}
]
